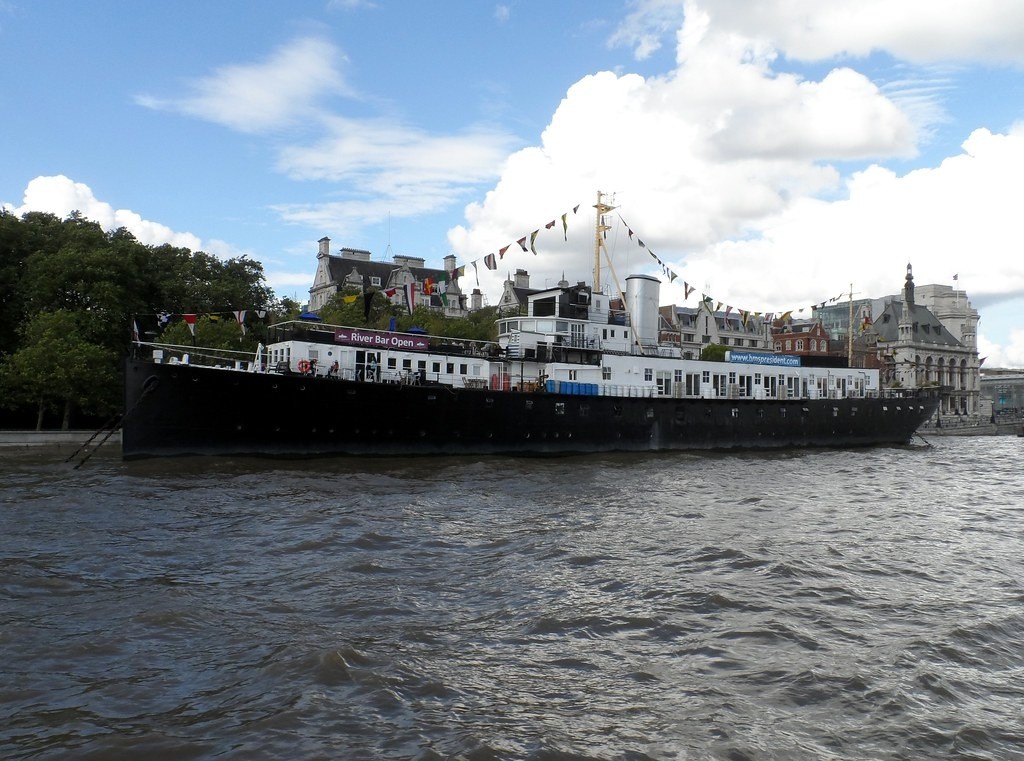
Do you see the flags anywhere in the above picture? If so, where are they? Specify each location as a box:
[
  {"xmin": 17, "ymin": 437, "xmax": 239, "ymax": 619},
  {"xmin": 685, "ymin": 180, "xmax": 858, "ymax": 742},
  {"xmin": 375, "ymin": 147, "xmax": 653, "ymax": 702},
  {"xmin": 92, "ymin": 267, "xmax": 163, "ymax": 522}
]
[
  {"xmin": 484, "ymin": 251, "xmax": 497, "ymax": 270},
  {"xmin": 451, "ymin": 265, "xmax": 465, "ymax": 281},
  {"xmin": 422, "ymin": 276, "xmax": 434, "ymax": 295},
  {"xmin": 763, "ymin": 312, "xmax": 775, "ymax": 325},
  {"xmin": 952, "ymin": 274, "xmax": 959, "ymax": 281},
  {"xmin": 403, "ymin": 283, "xmax": 415, "ymax": 316}
]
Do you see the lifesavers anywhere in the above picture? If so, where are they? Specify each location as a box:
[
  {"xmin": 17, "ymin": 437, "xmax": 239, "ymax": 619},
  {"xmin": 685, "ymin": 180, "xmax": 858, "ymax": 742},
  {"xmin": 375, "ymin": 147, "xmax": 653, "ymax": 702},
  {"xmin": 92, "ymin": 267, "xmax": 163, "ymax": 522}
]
[{"xmin": 297, "ymin": 358, "xmax": 311, "ymax": 373}]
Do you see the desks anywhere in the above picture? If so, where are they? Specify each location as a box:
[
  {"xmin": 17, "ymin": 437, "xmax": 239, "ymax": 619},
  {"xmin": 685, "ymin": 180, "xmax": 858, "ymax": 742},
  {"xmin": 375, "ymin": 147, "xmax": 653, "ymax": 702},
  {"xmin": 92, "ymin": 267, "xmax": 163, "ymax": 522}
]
[{"xmin": 468, "ymin": 378, "xmax": 484, "ymax": 387}]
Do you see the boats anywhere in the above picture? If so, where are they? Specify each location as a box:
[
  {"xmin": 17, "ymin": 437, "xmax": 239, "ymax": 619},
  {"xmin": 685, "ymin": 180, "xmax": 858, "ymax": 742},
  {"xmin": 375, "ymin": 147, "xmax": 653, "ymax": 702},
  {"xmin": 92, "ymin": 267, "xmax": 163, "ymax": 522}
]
[{"xmin": 122, "ymin": 287, "xmax": 945, "ymax": 463}]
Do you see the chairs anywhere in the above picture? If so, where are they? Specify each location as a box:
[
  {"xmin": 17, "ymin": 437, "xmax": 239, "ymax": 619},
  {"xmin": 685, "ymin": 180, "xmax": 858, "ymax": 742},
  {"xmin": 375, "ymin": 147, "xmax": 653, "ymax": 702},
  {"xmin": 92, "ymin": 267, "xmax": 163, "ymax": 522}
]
[{"xmin": 265, "ymin": 361, "xmax": 488, "ymax": 389}]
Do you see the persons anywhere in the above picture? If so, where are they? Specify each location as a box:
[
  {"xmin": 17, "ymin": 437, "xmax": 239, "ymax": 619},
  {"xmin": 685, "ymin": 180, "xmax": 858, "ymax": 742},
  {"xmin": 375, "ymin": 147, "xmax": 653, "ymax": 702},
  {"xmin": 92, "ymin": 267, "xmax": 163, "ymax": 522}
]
[
  {"xmin": 308, "ymin": 359, "xmax": 319, "ymax": 376},
  {"xmin": 332, "ymin": 359, "xmax": 340, "ymax": 368},
  {"xmin": 368, "ymin": 356, "xmax": 377, "ymax": 382}
]
